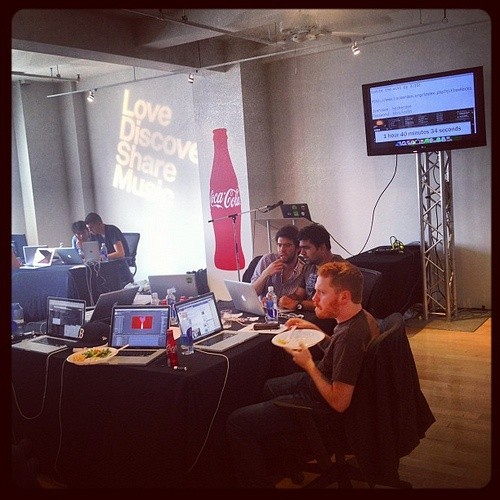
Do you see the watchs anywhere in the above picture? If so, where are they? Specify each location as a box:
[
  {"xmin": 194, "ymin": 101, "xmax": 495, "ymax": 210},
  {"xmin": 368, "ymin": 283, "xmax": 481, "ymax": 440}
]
[{"xmin": 295, "ymin": 300, "xmax": 304, "ymax": 311}]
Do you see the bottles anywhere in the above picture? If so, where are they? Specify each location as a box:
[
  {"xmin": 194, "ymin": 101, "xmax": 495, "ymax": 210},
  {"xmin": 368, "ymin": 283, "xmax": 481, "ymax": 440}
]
[
  {"xmin": 151, "ymin": 292, "xmax": 159, "ymax": 305},
  {"xmin": 11, "ymin": 303, "xmax": 24, "ymax": 336},
  {"xmin": 100, "ymin": 243, "xmax": 107, "ymax": 262},
  {"xmin": 162, "ymin": 300, "xmax": 166, "ymax": 305},
  {"xmin": 59, "ymin": 243, "xmax": 64, "ymax": 260},
  {"xmin": 266, "ymin": 286, "xmax": 279, "ymax": 324},
  {"xmin": 166, "ymin": 289, "xmax": 178, "ymax": 325},
  {"xmin": 180, "ymin": 296, "xmax": 186, "ymax": 302},
  {"xmin": 180, "ymin": 311, "xmax": 194, "ymax": 355},
  {"xmin": 165, "ymin": 329, "xmax": 178, "ymax": 367}
]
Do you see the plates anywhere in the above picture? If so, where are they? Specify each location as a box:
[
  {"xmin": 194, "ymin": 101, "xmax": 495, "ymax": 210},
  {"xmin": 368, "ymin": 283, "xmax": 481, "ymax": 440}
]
[
  {"xmin": 67, "ymin": 347, "xmax": 118, "ymax": 366},
  {"xmin": 271, "ymin": 329, "xmax": 325, "ymax": 349}
]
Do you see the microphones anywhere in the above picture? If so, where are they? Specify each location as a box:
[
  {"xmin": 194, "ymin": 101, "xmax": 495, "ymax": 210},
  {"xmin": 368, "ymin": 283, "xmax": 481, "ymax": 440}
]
[{"xmin": 262, "ymin": 200, "xmax": 284, "ymax": 213}]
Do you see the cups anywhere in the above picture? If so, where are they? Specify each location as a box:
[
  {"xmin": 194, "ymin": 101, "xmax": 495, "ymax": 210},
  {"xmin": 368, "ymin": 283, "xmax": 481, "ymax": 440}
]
[{"xmin": 220, "ymin": 309, "xmax": 232, "ymax": 329}]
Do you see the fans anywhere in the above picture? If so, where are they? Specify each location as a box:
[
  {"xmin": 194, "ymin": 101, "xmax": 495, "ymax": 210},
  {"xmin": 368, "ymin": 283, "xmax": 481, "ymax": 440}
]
[{"xmin": 206, "ymin": 9, "xmax": 394, "ymax": 62}]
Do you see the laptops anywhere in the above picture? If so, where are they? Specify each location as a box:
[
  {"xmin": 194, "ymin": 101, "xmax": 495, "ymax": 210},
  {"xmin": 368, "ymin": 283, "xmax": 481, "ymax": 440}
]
[
  {"xmin": 148, "ymin": 275, "xmax": 197, "ymax": 299},
  {"xmin": 84, "ymin": 286, "xmax": 140, "ymax": 323},
  {"xmin": 10, "ymin": 233, "xmax": 99, "ymax": 271},
  {"xmin": 91, "ymin": 304, "xmax": 171, "ymax": 367},
  {"xmin": 172, "ymin": 290, "xmax": 260, "ymax": 353},
  {"xmin": 224, "ymin": 279, "xmax": 296, "ymax": 316},
  {"xmin": 11, "ymin": 295, "xmax": 87, "ymax": 357}
]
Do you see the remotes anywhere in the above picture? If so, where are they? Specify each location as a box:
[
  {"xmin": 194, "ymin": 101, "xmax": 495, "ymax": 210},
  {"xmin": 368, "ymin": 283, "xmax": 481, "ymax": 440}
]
[{"xmin": 254, "ymin": 323, "xmax": 281, "ymax": 330}]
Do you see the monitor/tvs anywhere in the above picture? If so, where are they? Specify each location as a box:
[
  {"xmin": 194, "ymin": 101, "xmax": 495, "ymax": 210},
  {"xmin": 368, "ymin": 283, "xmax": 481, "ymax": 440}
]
[{"xmin": 361, "ymin": 64, "xmax": 487, "ymax": 156}]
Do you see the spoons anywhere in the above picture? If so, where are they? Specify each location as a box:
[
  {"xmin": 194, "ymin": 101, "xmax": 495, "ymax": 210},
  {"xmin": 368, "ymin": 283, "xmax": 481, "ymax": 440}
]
[{"xmin": 280, "ymin": 325, "xmax": 298, "ymax": 343}]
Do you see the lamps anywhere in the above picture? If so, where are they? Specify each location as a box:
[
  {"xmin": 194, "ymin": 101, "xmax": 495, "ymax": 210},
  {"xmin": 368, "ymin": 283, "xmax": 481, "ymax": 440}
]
[
  {"xmin": 351, "ymin": 40, "xmax": 360, "ymax": 55},
  {"xmin": 86, "ymin": 90, "xmax": 95, "ymax": 102},
  {"xmin": 188, "ymin": 74, "xmax": 194, "ymax": 83}
]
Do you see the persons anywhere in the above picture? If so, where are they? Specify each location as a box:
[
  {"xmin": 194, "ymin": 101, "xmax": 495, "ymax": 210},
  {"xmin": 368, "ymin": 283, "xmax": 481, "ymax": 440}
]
[
  {"xmin": 277, "ymin": 223, "xmax": 349, "ymax": 312},
  {"xmin": 224, "ymin": 262, "xmax": 383, "ymax": 500},
  {"xmin": 72, "ymin": 220, "xmax": 92, "ymax": 258},
  {"xmin": 85, "ymin": 212, "xmax": 130, "ymax": 259},
  {"xmin": 250, "ymin": 223, "xmax": 308, "ymax": 303}
]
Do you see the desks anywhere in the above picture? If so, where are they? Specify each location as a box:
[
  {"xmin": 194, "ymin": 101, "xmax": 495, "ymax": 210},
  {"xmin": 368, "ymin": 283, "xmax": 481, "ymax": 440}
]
[
  {"xmin": 12, "ymin": 300, "xmax": 333, "ymax": 490},
  {"xmin": 11, "ymin": 258, "xmax": 118, "ymax": 321},
  {"xmin": 346, "ymin": 246, "xmax": 423, "ymax": 320}
]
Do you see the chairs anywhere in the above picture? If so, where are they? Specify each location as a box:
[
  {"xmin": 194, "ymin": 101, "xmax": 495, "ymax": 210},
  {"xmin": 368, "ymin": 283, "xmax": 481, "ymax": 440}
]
[
  {"xmin": 361, "ymin": 268, "xmax": 382, "ymax": 315},
  {"xmin": 121, "ymin": 233, "xmax": 139, "ymax": 276},
  {"xmin": 275, "ymin": 400, "xmax": 413, "ymax": 490}
]
